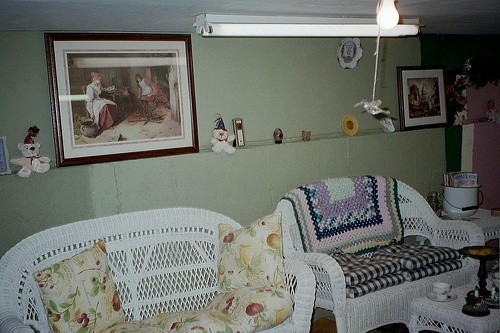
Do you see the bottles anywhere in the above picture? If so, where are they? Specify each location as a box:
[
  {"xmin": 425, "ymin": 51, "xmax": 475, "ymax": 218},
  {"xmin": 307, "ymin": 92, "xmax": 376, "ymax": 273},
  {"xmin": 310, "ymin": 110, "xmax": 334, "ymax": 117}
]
[{"xmin": 428, "ymin": 191, "xmax": 440, "ymax": 214}]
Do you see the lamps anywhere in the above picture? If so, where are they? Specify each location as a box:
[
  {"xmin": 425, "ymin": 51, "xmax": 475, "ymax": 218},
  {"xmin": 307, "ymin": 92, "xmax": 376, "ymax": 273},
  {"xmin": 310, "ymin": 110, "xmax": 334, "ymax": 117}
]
[
  {"xmin": 395, "ymin": 64, "xmax": 449, "ymax": 133},
  {"xmin": 195, "ymin": 13, "xmax": 422, "ymax": 39}
]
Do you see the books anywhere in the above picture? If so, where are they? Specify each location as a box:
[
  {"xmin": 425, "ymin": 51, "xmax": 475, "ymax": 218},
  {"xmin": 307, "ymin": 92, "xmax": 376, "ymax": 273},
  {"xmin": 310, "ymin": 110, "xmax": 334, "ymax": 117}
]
[{"xmin": 442, "ymin": 171, "xmax": 479, "ymax": 186}]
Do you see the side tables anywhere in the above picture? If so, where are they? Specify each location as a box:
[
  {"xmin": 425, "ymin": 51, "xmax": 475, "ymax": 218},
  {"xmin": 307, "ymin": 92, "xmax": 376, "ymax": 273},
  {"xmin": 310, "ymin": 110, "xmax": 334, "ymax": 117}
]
[{"xmin": 438, "ymin": 208, "xmax": 500, "ymax": 244}]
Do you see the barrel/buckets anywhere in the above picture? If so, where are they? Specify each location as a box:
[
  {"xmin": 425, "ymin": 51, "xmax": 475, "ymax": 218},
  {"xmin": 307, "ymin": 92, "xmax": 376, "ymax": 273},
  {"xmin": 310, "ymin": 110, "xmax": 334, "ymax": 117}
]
[{"xmin": 441, "ymin": 183, "xmax": 481, "ymax": 217}]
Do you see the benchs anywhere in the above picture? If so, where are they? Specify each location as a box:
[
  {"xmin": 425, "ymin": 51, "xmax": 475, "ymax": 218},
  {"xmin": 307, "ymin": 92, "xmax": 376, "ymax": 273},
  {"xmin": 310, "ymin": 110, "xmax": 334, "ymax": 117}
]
[
  {"xmin": 0, "ymin": 206, "xmax": 318, "ymax": 333},
  {"xmin": 279, "ymin": 174, "xmax": 488, "ymax": 333}
]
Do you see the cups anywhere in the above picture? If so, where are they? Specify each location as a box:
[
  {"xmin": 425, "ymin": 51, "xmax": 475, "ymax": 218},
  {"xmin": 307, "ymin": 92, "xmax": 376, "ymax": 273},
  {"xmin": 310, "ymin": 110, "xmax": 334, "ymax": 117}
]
[{"xmin": 433, "ymin": 282, "xmax": 451, "ymax": 300}]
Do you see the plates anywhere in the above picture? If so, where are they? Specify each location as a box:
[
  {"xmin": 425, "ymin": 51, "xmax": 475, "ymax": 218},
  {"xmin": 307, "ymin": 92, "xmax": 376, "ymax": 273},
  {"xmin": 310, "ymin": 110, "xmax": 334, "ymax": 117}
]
[{"xmin": 427, "ymin": 291, "xmax": 457, "ymax": 302}]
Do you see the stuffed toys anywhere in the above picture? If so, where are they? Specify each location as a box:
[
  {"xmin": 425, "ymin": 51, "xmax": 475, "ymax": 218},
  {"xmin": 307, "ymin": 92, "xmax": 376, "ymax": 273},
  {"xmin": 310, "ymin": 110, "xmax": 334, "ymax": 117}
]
[
  {"xmin": 10, "ymin": 134, "xmax": 52, "ymax": 177},
  {"xmin": 211, "ymin": 118, "xmax": 238, "ymax": 155}
]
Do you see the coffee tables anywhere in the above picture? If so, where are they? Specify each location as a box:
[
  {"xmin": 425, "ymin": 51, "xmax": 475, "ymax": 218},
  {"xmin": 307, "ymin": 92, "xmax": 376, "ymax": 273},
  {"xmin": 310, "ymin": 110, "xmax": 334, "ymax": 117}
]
[{"xmin": 409, "ymin": 280, "xmax": 500, "ymax": 333}]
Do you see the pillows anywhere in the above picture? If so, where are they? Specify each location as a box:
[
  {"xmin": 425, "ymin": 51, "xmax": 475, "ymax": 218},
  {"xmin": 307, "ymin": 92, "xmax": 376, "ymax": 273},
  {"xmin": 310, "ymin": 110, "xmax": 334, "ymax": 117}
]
[
  {"xmin": 32, "ymin": 238, "xmax": 126, "ymax": 333},
  {"xmin": 202, "ymin": 287, "xmax": 294, "ymax": 333},
  {"xmin": 142, "ymin": 309, "xmax": 238, "ymax": 333},
  {"xmin": 216, "ymin": 212, "xmax": 286, "ymax": 294},
  {"xmin": 100, "ymin": 321, "xmax": 167, "ymax": 333}
]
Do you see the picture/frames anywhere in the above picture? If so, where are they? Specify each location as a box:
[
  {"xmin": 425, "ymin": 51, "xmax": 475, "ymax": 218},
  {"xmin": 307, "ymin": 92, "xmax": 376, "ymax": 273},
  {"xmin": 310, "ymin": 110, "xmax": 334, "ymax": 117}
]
[{"xmin": 43, "ymin": 31, "xmax": 201, "ymax": 169}]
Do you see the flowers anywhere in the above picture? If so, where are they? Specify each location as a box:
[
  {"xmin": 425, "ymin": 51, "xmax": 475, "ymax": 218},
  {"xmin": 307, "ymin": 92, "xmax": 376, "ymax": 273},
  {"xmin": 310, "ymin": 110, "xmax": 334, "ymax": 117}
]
[{"xmin": 444, "ymin": 59, "xmax": 473, "ymax": 125}]
